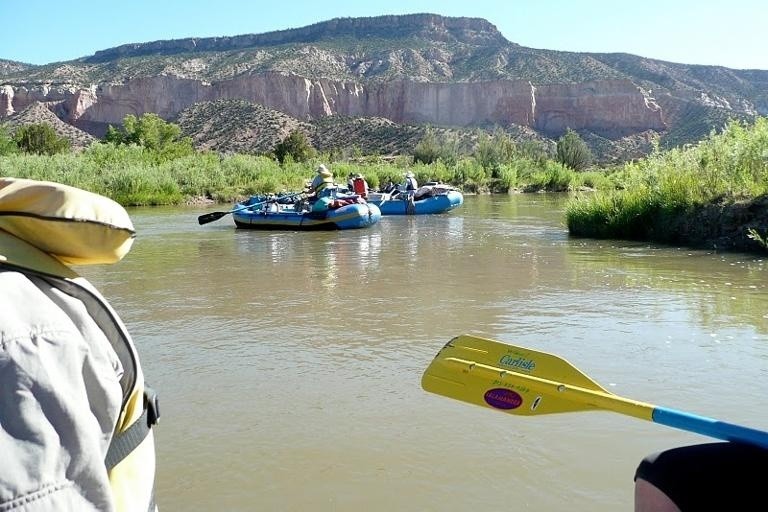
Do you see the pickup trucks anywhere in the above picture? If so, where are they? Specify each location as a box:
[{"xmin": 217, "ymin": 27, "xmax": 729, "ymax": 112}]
[{"xmin": 422, "ymin": 182, "xmax": 452, "ymax": 190}]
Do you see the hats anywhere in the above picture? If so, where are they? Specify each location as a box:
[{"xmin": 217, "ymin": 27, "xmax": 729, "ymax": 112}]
[
  {"xmin": 317, "ymin": 164, "xmax": 329, "ymax": 173},
  {"xmin": 349, "ymin": 172, "xmax": 363, "ymax": 180},
  {"xmin": 407, "ymin": 170, "xmax": 415, "ymax": 178}
]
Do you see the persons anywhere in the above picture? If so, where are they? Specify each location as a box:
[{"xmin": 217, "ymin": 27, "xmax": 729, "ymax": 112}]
[
  {"xmin": 396, "ymin": 171, "xmax": 418, "ymax": 196},
  {"xmin": 353, "ymin": 173, "xmax": 369, "ymax": 198},
  {"xmin": 0, "ymin": 174, "xmax": 161, "ymax": 512},
  {"xmin": 348, "ymin": 172, "xmax": 355, "ymax": 191},
  {"xmin": 302, "ymin": 164, "xmax": 334, "ymax": 204}
]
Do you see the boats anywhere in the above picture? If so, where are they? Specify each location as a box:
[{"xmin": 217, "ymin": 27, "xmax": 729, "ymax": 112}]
[{"xmin": 232, "ymin": 191, "xmax": 463, "ymax": 231}]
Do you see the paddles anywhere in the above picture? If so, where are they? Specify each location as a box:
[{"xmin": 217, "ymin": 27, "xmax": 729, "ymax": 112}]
[
  {"xmin": 198, "ymin": 192, "xmax": 305, "ymax": 225},
  {"xmin": 421, "ymin": 334, "xmax": 768, "ymax": 450}
]
